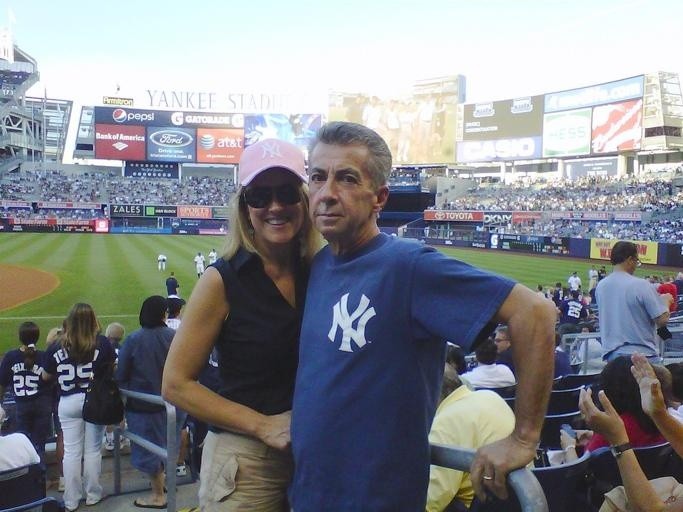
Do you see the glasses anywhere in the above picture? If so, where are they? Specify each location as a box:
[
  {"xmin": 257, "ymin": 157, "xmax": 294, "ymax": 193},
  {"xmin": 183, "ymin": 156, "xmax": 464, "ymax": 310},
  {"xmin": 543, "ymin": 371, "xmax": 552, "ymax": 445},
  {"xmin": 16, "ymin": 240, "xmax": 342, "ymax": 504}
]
[
  {"xmin": 494, "ymin": 337, "xmax": 509, "ymax": 343},
  {"xmin": 626, "ymin": 254, "xmax": 642, "ymax": 268},
  {"xmin": 239, "ymin": 182, "xmax": 304, "ymax": 208}
]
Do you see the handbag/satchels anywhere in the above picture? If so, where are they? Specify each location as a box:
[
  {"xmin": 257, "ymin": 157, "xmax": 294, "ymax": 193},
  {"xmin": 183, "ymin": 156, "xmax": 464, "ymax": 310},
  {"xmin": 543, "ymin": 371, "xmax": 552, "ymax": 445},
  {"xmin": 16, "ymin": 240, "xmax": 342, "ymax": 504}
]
[{"xmin": 79, "ymin": 374, "xmax": 127, "ymax": 426}]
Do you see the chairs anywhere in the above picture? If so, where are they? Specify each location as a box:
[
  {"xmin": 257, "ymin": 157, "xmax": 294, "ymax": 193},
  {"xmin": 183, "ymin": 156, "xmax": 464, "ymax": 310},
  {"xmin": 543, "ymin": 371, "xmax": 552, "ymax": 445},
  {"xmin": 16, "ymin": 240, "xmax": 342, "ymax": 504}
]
[
  {"xmin": 464, "ymin": 307, "xmax": 683, "ymax": 509},
  {"xmin": 430, "ymin": 173, "xmax": 682, "ymax": 242},
  {"xmin": 0, "ymin": 160, "xmax": 238, "ymax": 220},
  {"xmin": 0, "ymin": 461, "xmax": 57, "ymax": 511}
]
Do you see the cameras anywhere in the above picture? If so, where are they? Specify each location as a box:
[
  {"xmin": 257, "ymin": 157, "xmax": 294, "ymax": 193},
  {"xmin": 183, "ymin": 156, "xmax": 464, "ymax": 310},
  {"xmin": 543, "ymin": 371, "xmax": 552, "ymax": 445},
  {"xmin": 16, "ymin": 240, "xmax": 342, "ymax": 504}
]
[{"xmin": 57, "ymin": 331, "xmax": 62, "ymax": 336}]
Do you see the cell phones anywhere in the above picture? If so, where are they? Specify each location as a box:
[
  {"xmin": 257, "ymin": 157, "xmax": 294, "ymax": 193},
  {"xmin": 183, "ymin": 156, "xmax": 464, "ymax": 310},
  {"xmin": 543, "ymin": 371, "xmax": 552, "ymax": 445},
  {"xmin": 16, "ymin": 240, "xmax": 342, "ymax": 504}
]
[{"xmin": 559, "ymin": 418, "xmax": 577, "ymax": 440}]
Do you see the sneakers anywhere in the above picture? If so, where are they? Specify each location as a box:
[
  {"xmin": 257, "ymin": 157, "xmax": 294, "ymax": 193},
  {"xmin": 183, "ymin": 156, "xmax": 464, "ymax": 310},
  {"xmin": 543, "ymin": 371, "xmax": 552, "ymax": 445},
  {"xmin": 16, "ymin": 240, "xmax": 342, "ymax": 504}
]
[
  {"xmin": 57, "ymin": 476, "xmax": 66, "ymax": 493},
  {"xmin": 101, "ymin": 430, "xmax": 129, "ymax": 451},
  {"xmin": 176, "ymin": 460, "xmax": 187, "ymax": 476}
]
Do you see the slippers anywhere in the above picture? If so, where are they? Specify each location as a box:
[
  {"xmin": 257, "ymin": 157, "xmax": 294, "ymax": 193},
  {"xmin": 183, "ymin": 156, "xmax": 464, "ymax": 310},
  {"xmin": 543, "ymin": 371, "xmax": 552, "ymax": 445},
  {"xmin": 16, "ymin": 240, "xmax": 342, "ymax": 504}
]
[{"xmin": 133, "ymin": 496, "xmax": 167, "ymax": 509}]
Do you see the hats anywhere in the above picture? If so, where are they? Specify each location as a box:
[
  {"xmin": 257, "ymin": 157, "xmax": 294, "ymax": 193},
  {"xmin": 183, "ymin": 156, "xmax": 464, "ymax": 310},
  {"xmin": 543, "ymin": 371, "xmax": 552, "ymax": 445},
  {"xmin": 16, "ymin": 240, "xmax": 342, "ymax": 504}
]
[{"xmin": 231, "ymin": 138, "xmax": 308, "ymax": 188}]
